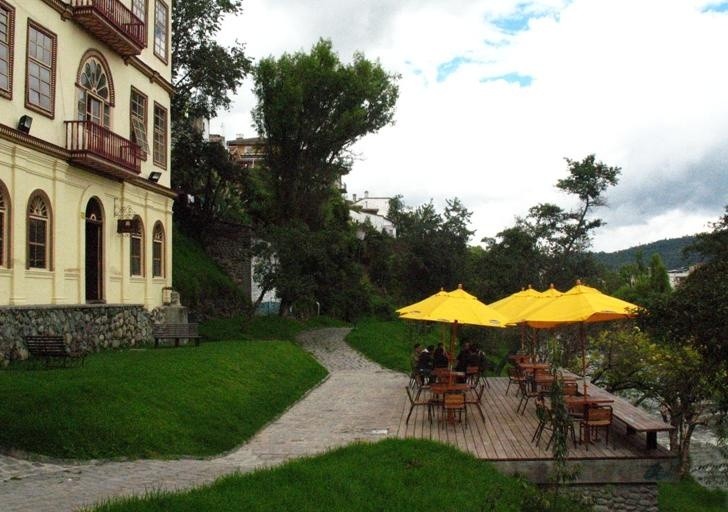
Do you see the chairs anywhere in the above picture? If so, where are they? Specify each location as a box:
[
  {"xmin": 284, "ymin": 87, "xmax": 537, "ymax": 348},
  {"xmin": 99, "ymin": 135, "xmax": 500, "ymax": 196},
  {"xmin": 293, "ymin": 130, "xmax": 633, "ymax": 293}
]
[{"xmin": 404, "ymin": 353, "xmax": 612, "ymax": 451}]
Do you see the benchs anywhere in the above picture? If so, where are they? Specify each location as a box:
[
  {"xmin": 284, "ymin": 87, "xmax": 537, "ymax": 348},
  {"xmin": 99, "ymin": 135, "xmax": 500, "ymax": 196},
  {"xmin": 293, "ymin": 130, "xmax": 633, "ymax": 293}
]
[
  {"xmin": 577, "ymin": 379, "xmax": 675, "ymax": 451},
  {"xmin": 21, "ymin": 333, "xmax": 88, "ymax": 369},
  {"xmin": 148, "ymin": 321, "xmax": 203, "ymax": 350}
]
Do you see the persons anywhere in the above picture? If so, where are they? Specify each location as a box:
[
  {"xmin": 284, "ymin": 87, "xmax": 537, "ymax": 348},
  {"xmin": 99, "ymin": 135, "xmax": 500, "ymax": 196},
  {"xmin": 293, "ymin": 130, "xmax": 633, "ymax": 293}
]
[{"xmin": 411, "ymin": 337, "xmax": 486, "ymax": 385}]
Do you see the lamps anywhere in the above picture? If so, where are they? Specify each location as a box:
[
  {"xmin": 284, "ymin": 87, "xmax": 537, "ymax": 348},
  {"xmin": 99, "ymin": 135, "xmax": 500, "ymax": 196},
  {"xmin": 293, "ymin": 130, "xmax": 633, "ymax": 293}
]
[
  {"xmin": 146, "ymin": 170, "xmax": 162, "ymax": 183},
  {"xmin": 16, "ymin": 115, "xmax": 33, "ymax": 133}
]
